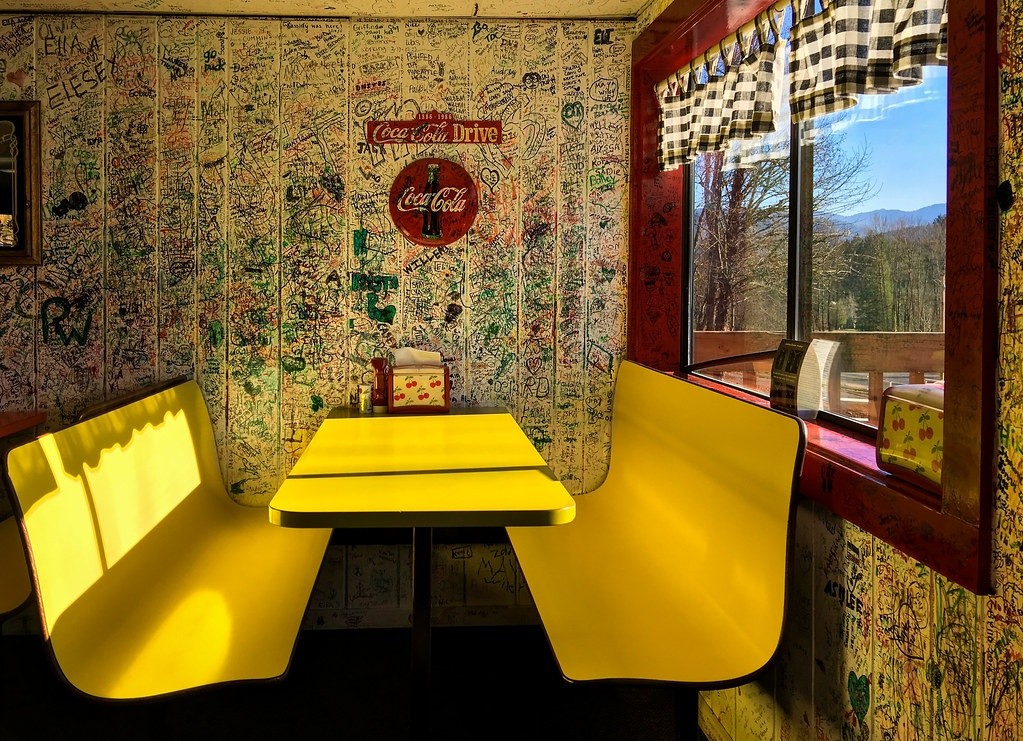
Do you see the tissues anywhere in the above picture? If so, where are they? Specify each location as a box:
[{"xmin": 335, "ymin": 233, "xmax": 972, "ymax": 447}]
[
  {"xmin": 387, "ymin": 346, "xmax": 451, "ymax": 414},
  {"xmin": 875, "ymin": 379, "xmax": 944, "ymax": 495}
]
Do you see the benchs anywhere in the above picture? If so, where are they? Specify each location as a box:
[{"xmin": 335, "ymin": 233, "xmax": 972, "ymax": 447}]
[
  {"xmin": 500, "ymin": 356, "xmax": 809, "ymax": 741},
  {"xmin": 6, "ymin": 379, "xmax": 334, "ymax": 741}
]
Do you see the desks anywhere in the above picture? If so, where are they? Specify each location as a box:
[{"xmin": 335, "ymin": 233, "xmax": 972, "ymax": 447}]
[{"xmin": 266, "ymin": 402, "xmax": 579, "ymax": 741}]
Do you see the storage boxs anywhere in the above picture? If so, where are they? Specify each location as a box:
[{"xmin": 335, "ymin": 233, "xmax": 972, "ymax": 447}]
[{"xmin": 877, "ymin": 382, "xmax": 944, "ymax": 497}]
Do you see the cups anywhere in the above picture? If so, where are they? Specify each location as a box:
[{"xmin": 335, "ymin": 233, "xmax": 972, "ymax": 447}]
[{"xmin": 358, "ymin": 385, "xmax": 373, "ymax": 414}]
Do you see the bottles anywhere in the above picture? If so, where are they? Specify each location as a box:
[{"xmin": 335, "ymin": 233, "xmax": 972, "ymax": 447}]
[
  {"xmin": 371, "ymin": 358, "xmax": 388, "ymax": 414},
  {"xmin": 421, "ymin": 163, "xmax": 444, "ymax": 240}
]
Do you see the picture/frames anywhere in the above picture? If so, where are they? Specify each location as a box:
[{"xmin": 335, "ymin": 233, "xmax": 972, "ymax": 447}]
[{"xmin": 0, "ymin": 99, "xmax": 44, "ymax": 267}]
